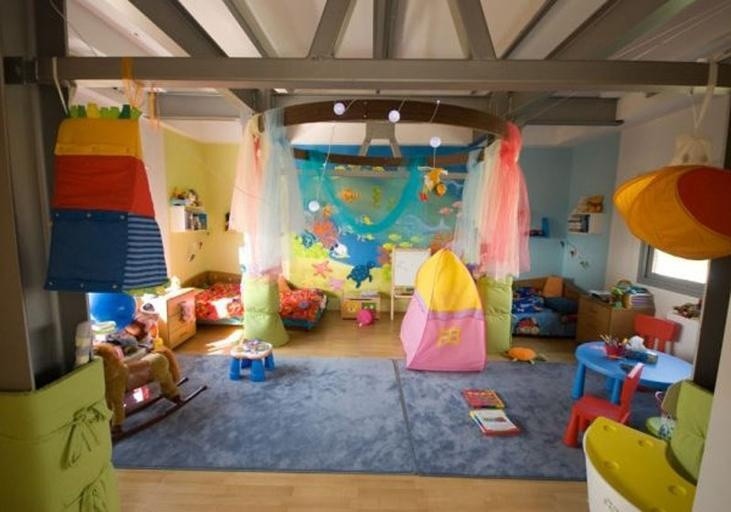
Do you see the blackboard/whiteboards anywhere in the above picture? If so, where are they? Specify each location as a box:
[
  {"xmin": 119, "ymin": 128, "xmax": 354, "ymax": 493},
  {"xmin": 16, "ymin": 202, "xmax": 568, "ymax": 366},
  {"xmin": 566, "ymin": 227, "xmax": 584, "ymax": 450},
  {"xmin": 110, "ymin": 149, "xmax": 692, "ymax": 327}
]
[{"xmin": 392, "ymin": 249, "xmax": 431, "ymax": 288}]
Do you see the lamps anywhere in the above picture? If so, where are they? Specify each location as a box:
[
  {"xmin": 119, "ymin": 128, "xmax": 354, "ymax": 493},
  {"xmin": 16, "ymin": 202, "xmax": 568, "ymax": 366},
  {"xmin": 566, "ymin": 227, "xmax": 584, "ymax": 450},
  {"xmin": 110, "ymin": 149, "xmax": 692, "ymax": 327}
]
[
  {"xmin": 308, "ymin": 200, "xmax": 320, "ymax": 212},
  {"xmin": 334, "ymin": 102, "xmax": 346, "ymax": 115},
  {"xmin": 388, "ymin": 96, "xmax": 407, "ymax": 123},
  {"xmin": 417, "ymin": 136, "xmax": 448, "ymax": 176}
]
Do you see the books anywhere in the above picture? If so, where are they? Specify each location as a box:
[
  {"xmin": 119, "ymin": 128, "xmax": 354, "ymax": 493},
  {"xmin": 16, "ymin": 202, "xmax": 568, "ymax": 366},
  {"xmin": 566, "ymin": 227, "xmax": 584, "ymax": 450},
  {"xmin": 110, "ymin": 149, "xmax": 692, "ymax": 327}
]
[{"xmin": 460, "ymin": 386, "xmax": 524, "ymax": 437}]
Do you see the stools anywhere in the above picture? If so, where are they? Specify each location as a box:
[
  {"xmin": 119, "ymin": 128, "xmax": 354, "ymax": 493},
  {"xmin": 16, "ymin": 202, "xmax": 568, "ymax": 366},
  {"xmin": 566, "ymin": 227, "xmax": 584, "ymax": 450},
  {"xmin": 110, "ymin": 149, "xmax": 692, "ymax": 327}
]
[{"xmin": 229, "ymin": 340, "xmax": 275, "ymax": 382}]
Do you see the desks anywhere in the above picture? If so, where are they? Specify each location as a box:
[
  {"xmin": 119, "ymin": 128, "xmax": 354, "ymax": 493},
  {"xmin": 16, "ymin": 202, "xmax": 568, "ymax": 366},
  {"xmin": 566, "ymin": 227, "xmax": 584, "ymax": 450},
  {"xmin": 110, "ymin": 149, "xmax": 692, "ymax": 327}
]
[{"xmin": 570, "ymin": 340, "xmax": 694, "ymax": 406}]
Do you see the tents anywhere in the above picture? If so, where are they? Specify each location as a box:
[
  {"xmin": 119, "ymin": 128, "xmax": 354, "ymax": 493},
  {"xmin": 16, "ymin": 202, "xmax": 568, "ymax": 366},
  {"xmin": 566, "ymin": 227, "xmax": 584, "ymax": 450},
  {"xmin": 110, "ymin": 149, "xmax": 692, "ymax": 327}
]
[{"xmin": 395, "ymin": 244, "xmax": 489, "ymax": 375}]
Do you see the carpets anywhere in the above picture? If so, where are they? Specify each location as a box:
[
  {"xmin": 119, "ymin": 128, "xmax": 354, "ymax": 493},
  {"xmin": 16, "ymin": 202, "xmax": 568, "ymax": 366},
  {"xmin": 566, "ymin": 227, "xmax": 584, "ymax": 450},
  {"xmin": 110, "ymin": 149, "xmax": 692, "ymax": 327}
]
[
  {"xmin": 112, "ymin": 352, "xmax": 419, "ymax": 475},
  {"xmin": 395, "ymin": 357, "xmax": 664, "ymax": 480}
]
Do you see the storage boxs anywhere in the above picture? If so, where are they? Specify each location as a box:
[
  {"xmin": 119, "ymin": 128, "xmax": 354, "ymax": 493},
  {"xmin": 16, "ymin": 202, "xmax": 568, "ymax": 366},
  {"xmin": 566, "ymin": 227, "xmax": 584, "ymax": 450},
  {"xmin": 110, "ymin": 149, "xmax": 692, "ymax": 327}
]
[{"xmin": 340, "ymin": 296, "xmax": 380, "ymax": 320}]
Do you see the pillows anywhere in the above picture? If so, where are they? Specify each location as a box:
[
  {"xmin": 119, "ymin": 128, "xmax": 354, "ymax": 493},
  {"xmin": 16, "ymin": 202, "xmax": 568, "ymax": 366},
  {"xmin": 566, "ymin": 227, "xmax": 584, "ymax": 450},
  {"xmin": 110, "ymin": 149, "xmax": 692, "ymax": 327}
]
[
  {"xmin": 545, "ymin": 297, "xmax": 578, "ymax": 316},
  {"xmin": 543, "ymin": 276, "xmax": 563, "ymax": 296}
]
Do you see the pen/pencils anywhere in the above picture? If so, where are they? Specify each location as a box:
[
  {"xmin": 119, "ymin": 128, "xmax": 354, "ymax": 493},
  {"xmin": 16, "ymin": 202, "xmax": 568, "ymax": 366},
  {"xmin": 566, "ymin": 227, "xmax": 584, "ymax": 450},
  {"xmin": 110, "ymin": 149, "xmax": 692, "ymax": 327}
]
[{"xmin": 600, "ymin": 334, "xmax": 620, "ymax": 346}]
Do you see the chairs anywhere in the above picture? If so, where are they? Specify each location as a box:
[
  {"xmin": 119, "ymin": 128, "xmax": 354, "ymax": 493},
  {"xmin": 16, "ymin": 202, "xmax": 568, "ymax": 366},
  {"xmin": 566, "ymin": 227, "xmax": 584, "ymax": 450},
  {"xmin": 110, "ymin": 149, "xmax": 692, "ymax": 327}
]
[
  {"xmin": 563, "ymin": 362, "xmax": 644, "ymax": 449},
  {"xmin": 633, "ymin": 314, "xmax": 681, "ymax": 352}
]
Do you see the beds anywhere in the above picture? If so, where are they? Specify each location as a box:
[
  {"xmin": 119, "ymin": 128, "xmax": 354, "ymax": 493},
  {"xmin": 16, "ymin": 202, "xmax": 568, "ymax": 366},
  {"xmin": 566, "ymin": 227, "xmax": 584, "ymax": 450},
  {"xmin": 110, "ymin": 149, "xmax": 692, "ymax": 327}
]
[
  {"xmin": 509, "ymin": 274, "xmax": 585, "ymax": 338},
  {"xmin": 181, "ymin": 271, "xmax": 327, "ymax": 331}
]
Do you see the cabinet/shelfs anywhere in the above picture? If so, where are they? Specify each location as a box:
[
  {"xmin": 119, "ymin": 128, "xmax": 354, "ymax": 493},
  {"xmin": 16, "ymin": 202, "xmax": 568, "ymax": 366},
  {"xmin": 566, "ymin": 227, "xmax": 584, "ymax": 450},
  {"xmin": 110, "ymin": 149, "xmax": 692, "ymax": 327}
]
[
  {"xmin": 566, "ymin": 212, "xmax": 605, "ymax": 235},
  {"xmin": 138, "ymin": 287, "xmax": 197, "ymax": 349},
  {"xmin": 576, "ymin": 294, "xmax": 654, "ymax": 342},
  {"xmin": 171, "ymin": 206, "xmax": 209, "ymax": 233}
]
